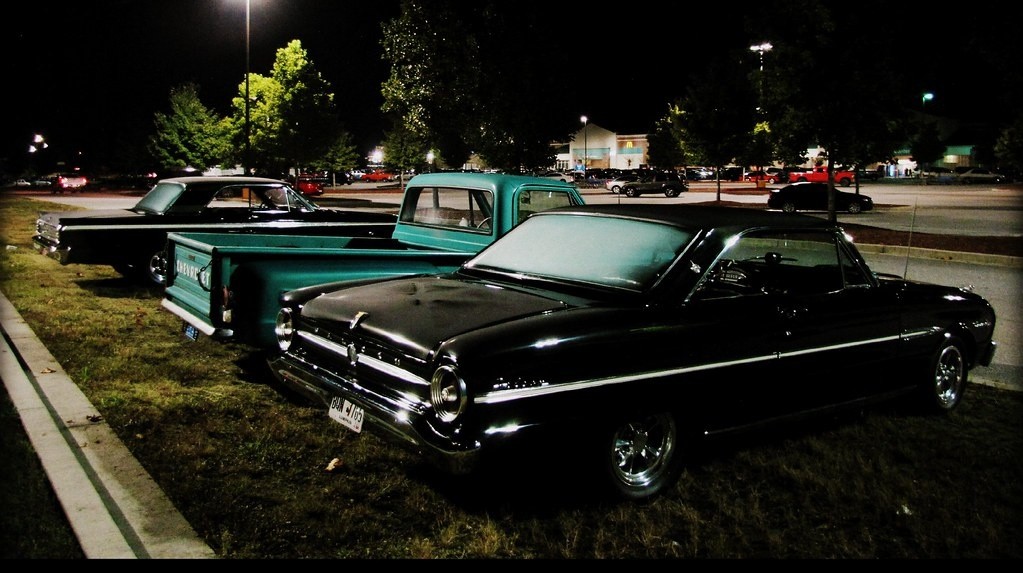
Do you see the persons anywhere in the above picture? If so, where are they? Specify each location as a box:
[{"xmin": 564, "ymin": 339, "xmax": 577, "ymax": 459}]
[{"xmin": 575, "ymin": 160, "xmax": 585, "ymax": 171}]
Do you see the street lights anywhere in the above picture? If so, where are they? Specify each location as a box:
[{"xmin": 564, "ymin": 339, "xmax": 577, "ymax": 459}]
[
  {"xmin": 240, "ymin": 0, "xmax": 261, "ymax": 174},
  {"xmin": 580, "ymin": 115, "xmax": 588, "ymax": 187},
  {"xmin": 748, "ymin": 42, "xmax": 773, "ymax": 188},
  {"xmin": 920, "ymin": 92, "xmax": 934, "ymax": 174}
]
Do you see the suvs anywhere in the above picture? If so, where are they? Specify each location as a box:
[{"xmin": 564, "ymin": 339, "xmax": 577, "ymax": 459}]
[{"xmin": 16, "ymin": 180, "xmax": 30, "ymax": 186}]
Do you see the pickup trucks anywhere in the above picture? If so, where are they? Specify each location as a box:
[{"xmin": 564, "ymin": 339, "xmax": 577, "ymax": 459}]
[{"xmin": 789, "ymin": 166, "xmax": 855, "ymax": 186}]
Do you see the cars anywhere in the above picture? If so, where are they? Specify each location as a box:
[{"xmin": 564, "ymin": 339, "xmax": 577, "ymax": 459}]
[
  {"xmin": 545, "ymin": 168, "xmax": 718, "ymax": 198},
  {"xmin": 49, "ymin": 170, "xmax": 86, "ymax": 193},
  {"xmin": 30, "ymin": 176, "xmax": 398, "ymax": 294},
  {"xmin": 285, "ymin": 172, "xmax": 323, "ymax": 196},
  {"xmin": 266, "ymin": 204, "xmax": 996, "ymax": 505},
  {"xmin": 31, "ymin": 180, "xmax": 51, "ymax": 186},
  {"xmin": 767, "ymin": 182, "xmax": 874, "ymax": 214},
  {"xmin": 327, "ymin": 169, "xmax": 367, "ymax": 185},
  {"xmin": 361, "ymin": 169, "xmax": 415, "ymax": 182},
  {"xmin": 739, "ymin": 167, "xmax": 783, "ymax": 184},
  {"xmin": 919, "ymin": 166, "xmax": 1006, "ymax": 184}
]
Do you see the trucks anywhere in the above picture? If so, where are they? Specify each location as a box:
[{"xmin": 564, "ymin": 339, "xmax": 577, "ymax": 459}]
[{"xmin": 161, "ymin": 174, "xmax": 585, "ymax": 344}]
